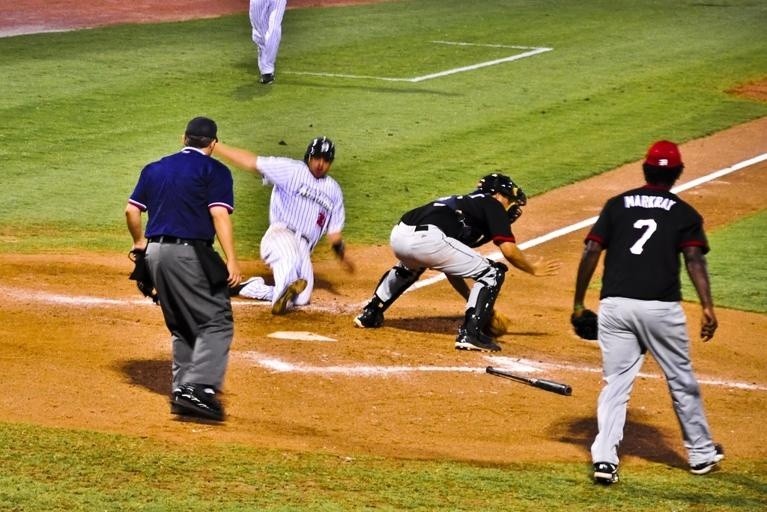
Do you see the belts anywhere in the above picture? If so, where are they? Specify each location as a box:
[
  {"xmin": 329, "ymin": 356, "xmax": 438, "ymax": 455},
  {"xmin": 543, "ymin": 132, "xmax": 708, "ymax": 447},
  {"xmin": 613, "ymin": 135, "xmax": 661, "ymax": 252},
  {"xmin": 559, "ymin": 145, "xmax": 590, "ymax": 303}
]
[
  {"xmin": 289, "ymin": 227, "xmax": 309, "ymax": 243},
  {"xmin": 148, "ymin": 236, "xmax": 193, "ymax": 246},
  {"xmin": 397, "ymin": 220, "xmax": 429, "ymax": 230}
]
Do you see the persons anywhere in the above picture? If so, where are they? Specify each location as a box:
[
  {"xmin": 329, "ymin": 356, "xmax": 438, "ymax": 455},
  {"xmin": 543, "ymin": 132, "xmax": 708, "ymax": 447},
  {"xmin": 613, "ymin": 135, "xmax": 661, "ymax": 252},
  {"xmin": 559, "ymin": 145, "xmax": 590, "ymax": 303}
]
[
  {"xmin": 571, "ymin": 140, "xmax": 725, "ymax": 485},
  {"xmin": 212, "ymin": 136, "xmax": 355, "ymax": 316},
  {"xmin": 353, "ymin": 172, "xmax": 562, "ymax": 353},
  {"xmin": 125, "ymin": 116, "xmax": 241, "ymax": 421},
  {"xmin": 248, "ymin": 0, "xmax": 288, "ymax": 83}
]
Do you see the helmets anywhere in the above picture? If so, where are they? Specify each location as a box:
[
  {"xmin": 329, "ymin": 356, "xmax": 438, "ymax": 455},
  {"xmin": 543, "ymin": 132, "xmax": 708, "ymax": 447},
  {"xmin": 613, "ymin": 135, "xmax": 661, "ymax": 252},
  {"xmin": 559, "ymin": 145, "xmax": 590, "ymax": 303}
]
[
  {"xmin": 304, "ymin": 138, "xmax": 336, "ymax": 164},
  {"xmin": 478, "ymin": 172, "xmax": 526, "ymax": 224}
]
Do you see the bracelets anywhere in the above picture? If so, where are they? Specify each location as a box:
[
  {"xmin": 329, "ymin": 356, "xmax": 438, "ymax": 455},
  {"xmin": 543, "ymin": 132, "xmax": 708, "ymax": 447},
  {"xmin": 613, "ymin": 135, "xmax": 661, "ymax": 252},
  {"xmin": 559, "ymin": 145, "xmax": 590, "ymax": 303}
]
[
  {"xmin": 574, "ymin": 303, "xmax": 584, "ymax": 311},
  {"xmin": 332, "ymin": 240, "xmax": 345, "ymax": 256}
]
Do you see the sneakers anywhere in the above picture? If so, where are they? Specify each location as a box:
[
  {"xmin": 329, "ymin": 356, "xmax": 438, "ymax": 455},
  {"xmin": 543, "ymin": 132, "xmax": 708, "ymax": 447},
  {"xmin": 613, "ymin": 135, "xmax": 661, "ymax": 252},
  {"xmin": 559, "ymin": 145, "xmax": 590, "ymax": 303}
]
[
  {"xmin": 272, "ymin": 279, "xmax": 307, "ymax": 315},
  {"xmin": 691, "ymin": 445, "xmax": 724, "ymax": 474},
  {"xmin": 171, "ymin": 383, "xmax": 225, "ymax": 422},
  {"xmin": 259, "ymin": 73, "xmax": 274, "ymax": 84},
  {"xmin": 228, "ymin": 277, "xmax": 264, "ymax": 297},
  {"xmin": 592, "ymin": 462, "xmax": 619, "ymax": 483},
  {"xmin": 454, "ymin": 329, "xmax": 501, "ymax": 353},
  {"xmin": 354, "ymin": 311, "xmax": 383, "ymax": 327}
]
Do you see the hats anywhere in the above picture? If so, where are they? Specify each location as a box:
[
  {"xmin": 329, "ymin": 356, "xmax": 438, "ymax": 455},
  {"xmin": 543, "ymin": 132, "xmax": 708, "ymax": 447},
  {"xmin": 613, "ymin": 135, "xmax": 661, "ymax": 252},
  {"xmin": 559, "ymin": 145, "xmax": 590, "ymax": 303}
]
[
  {"xmin": 645, "ymin": 140, "xmax": 681, "ymax": 167},
  {"xmin": 186, "ymin": 117, "xmax": 219, "ymax": 141}
]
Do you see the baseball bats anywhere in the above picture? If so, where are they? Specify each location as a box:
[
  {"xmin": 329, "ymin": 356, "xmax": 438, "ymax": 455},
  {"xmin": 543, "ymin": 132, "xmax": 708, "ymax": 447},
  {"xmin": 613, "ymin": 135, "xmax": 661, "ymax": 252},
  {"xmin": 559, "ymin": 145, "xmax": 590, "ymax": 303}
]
[{"xmin": 486, "ymin": 366, "xmax": 571, "ymax": 395}]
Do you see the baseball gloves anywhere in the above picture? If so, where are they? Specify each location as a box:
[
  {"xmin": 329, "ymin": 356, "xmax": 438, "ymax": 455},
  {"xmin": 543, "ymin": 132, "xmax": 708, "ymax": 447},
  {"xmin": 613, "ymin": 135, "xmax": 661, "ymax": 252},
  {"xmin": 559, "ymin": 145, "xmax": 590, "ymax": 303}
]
[
  {"xmin": 490, "ymin": 311, "xmax": 510, "ymax": 336},
  {"xmin": 571, "ymin": 309, "xmax": 598, "ymax": 340}
]
